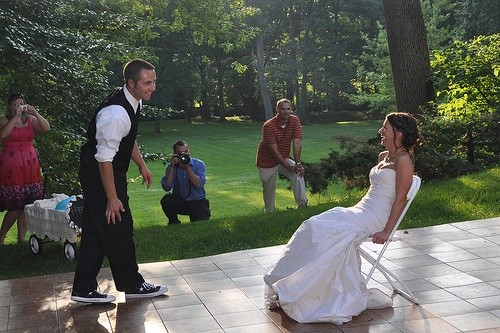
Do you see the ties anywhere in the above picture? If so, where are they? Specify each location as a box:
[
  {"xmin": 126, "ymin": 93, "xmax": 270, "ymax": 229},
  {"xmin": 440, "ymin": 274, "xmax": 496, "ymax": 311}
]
[{"xmin": 136, "ymin": 102, "xmax": 140, "ymax": 134}]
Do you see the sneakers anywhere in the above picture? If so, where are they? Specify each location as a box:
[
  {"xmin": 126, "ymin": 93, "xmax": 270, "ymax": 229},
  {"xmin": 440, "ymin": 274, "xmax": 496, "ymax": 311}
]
[
  {"xmin": 70, "ymin": 289, "xmax": 116, "ymax": 303},
  {"xmin": 125, "ymin": 282, "xmax": 169, "ymax": 297}
]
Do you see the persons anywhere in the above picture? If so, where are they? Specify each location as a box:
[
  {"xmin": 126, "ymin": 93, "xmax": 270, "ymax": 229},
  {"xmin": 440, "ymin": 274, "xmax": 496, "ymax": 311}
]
[
  {"xmin": 70, "ymin": 59, "xmax": 167, "ymax": 303},
  {"xmin": 263, "ymin": 113, "xmax": 418, "ymax": 325},
  {"xmin": 0, "ymin": 92, "xmax": 50, "ymax": 246},
  {"xmin": 256, "ymin": 98, "xmax": 308, "ymax": 210},
  {"xmin": 159, "ymin": 140, "xmax": 210, "ymax": 225}
]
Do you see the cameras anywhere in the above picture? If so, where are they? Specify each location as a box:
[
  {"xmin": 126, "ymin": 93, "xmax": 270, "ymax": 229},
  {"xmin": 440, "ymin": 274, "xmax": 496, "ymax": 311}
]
[
  {"xmin": 176, "ymin": 152, "xmax": 191, "ymax": 164},
  {"xmin": 19, "ymin": 105, "xmax": 27, "ymax": 111}
]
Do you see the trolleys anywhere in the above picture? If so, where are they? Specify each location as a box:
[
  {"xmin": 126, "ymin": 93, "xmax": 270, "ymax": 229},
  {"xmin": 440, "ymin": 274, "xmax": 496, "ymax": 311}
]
[{"xmin": 24, "ymin": 166, "xmax": 84, "ymax": 260}]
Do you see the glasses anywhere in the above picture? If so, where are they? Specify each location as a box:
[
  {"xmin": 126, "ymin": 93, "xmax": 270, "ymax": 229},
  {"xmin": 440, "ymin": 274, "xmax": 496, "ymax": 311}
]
[{"xmin": 10, "ymin": 93, "xmax": 24, "ymax": 98}]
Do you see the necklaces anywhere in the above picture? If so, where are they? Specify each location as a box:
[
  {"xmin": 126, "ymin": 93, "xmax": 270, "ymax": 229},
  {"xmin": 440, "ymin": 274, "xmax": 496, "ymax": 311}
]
[{"xmin": 385, "ymin": 149, "xmax": 403, "ymax": 162}]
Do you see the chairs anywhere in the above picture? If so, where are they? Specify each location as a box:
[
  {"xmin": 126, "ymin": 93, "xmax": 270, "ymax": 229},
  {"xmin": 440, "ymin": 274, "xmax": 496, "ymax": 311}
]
[{"xmin": 355, "ymin": 175, "xmax": 421, "ymax": 304}]
